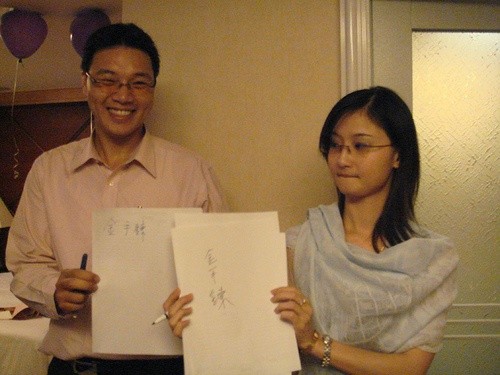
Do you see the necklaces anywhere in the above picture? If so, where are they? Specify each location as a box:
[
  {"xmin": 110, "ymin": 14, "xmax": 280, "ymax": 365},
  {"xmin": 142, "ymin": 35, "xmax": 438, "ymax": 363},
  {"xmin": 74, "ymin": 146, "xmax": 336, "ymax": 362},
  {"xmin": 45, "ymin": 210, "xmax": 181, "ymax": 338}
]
[{"xmin": 379, "ymin": 247, "xmax": 385, "ymax": 252}]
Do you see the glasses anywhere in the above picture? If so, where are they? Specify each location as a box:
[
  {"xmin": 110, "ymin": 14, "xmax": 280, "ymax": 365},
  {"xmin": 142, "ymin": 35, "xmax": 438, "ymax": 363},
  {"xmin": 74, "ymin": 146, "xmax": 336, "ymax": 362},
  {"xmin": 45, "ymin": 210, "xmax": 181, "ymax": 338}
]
[
  {"xmin": 85, "ymin": 71, "xmax": 156, "ymax": 96},
  {"xmin": 321, "ymin": 138, "xmax": 394, "ymax": 158}
]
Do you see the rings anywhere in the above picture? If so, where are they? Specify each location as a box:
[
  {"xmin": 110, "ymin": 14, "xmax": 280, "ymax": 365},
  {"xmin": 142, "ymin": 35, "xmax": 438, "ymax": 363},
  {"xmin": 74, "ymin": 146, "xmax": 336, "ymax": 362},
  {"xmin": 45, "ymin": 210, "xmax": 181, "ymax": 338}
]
[{"xmin": 301, "ymin": 299, "xmax": 305, "ymax": 305}]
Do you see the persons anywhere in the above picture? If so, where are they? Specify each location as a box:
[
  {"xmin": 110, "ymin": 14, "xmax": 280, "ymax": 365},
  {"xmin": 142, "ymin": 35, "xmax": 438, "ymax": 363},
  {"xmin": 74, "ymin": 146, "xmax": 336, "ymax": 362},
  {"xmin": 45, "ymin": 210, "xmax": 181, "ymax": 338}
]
[
  {"xmin": 163, "ymin": 87, "xmax": 457, "ymax": 375},
  {"xmin": 6, "ymin": 22, "xmax": 227, "ymax": 375}
]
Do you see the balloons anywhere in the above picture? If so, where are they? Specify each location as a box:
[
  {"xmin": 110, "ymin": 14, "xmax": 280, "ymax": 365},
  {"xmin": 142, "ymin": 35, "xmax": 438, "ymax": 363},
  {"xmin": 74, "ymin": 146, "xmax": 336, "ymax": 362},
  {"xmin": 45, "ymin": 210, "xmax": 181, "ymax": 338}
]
[
  {"xmin": 70, "ymin": 12, "xmax": 111, "ymax": 59},
  {"xmin": 0, "ymin": 10, "xmax": 48, "ymax": 63}
]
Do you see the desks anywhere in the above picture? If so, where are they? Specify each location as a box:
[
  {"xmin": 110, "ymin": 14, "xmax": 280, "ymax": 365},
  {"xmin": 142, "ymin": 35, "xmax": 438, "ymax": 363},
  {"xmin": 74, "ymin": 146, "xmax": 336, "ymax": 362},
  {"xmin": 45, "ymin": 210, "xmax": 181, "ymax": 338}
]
[{"xmin": 0, "ymin": 272, "xmax": 55, "ymax": 375}]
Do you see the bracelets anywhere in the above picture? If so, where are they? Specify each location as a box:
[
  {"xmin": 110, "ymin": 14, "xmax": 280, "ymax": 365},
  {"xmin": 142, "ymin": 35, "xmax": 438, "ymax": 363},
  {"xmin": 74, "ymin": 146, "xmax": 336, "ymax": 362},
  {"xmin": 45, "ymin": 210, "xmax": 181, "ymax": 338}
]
[
  {"xmin": 322, "ymin": 335, "xmax": 332, "ymax": 367},
  {"xmin": 304, "ymin": 330, "xmax": 319, "ymax": 350}
]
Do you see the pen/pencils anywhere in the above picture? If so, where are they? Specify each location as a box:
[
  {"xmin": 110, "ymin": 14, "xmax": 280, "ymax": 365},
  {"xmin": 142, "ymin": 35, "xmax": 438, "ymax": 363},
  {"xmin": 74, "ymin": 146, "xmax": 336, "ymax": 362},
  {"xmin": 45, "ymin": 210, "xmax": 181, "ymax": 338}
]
[
  {"xmin": 151, "ymin": 311, "xmax": 168, "ymax": 326},
  {"xmin": 72, "ymin": 253, "xmax": 87, "ymax": 319}
]
